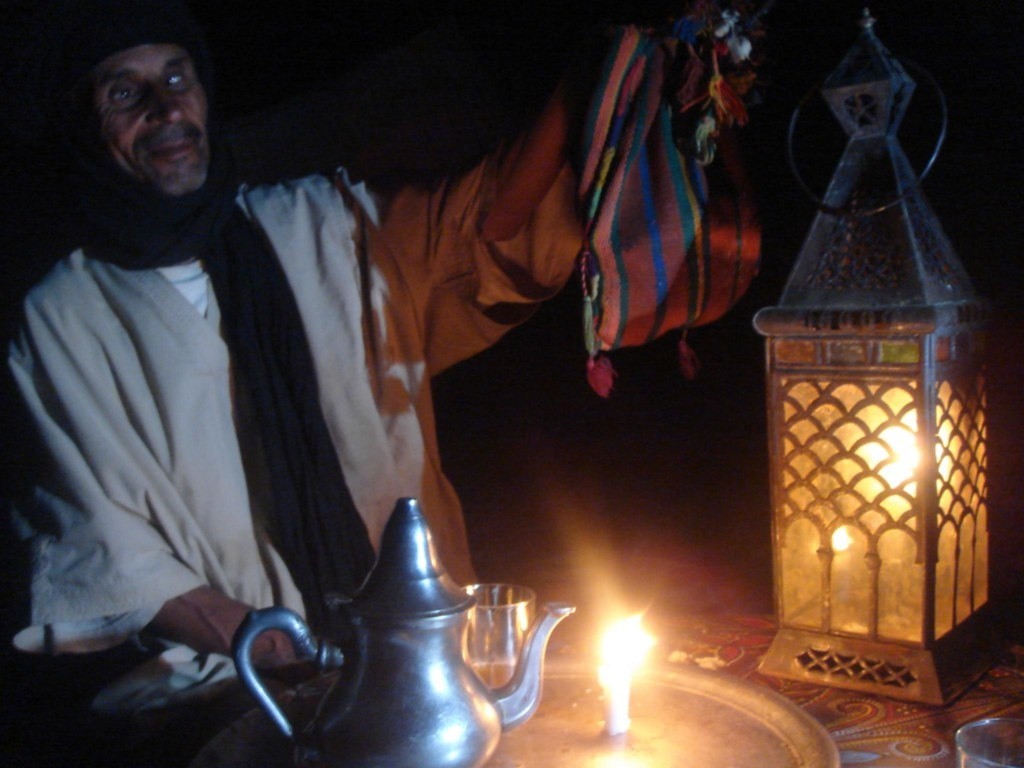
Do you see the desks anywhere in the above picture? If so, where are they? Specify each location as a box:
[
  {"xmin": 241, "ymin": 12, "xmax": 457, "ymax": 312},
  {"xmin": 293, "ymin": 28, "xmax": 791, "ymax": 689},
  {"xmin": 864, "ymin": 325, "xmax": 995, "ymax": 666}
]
[{"xmin": 190, "ymin": 610, "xmax": 1024, "ymax": 768}]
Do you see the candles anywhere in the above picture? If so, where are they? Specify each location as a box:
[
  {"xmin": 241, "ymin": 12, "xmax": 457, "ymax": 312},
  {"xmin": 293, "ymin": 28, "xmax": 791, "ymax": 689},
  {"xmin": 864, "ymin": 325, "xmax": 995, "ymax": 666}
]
[{"xmin": 592, "ymin": 609, "xmax": 652, "ymax": 735}]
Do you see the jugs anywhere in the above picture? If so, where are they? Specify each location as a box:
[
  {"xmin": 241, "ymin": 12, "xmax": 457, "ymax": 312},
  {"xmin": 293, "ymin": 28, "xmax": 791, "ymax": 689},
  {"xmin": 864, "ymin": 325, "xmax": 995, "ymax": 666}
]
[{"xmin": 230, "ymin": 496, "xmax": 577, "ymax": 768}]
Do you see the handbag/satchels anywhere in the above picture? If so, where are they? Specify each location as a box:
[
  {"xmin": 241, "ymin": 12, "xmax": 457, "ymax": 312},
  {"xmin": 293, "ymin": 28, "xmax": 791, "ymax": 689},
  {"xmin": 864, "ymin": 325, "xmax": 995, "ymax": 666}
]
[{"xmin": 578, "ymin": 1, "xmax": 771, "ymax": 400}]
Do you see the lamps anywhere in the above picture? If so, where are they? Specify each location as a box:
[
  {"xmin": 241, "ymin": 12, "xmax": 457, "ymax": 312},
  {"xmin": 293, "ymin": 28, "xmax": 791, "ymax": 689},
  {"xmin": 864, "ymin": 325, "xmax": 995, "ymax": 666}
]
[{"xmin": 753, "ymin": 6, "xmax": 1013, "ymax": 711}]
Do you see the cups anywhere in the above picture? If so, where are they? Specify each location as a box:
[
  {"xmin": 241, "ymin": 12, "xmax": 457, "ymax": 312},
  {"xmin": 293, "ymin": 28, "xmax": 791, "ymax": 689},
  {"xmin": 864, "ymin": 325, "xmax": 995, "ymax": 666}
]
[
  {"xmin": 955, "ymin": 718, "xmax": 1024, "ymax": 768},
  {"xmin": 458, "ymin": 583, "xmax": 538, "ymax": 691}
]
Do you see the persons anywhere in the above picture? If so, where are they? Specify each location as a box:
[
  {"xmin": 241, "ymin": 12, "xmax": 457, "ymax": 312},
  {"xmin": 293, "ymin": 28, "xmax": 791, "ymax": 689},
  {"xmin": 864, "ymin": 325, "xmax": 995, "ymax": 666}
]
[{"xmin": 0, "ymin": 0, "xmax": 582, "ymax": 768}]
